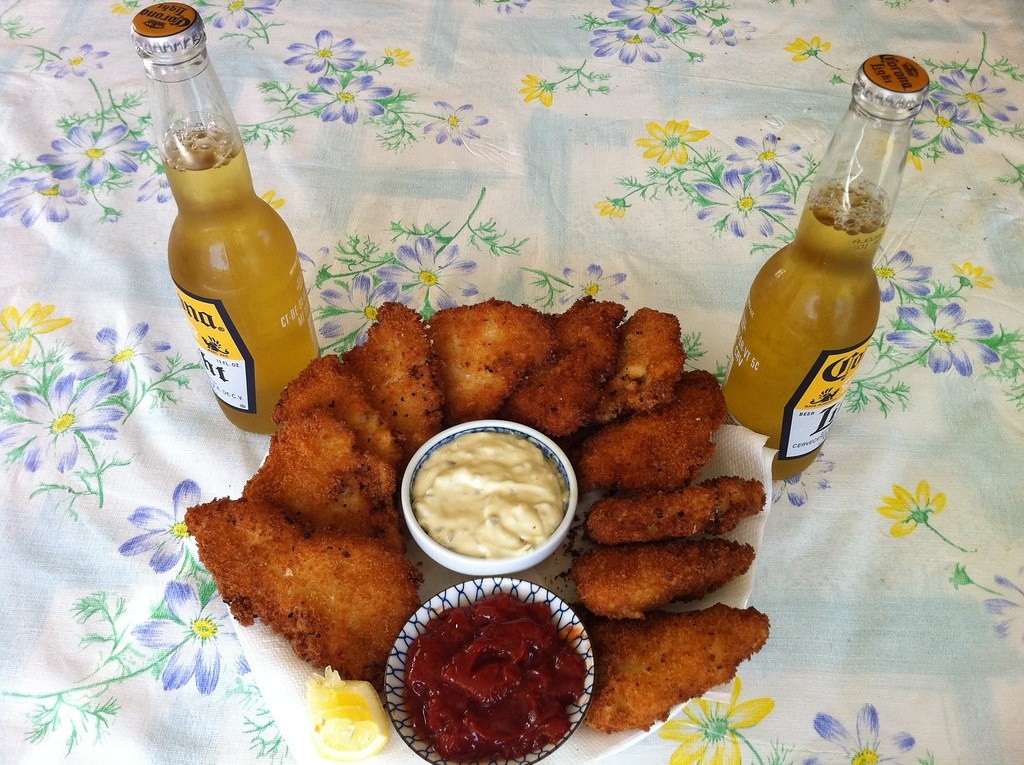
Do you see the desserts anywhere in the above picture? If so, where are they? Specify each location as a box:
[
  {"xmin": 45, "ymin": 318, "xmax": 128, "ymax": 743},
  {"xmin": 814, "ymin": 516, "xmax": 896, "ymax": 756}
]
[{"xmin": 412, "ymin": 431, "xmax": 566, "ymax": 560}]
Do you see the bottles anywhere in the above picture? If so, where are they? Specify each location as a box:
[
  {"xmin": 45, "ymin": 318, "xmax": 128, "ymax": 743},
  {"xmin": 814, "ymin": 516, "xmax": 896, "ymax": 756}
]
[
  {"xmin": 130, "ymin": 4, "xmax": 320, "ymax": 433},
  {"xmin": 723, "ymin": 54, "xmax": 930, "ymax": 481}
]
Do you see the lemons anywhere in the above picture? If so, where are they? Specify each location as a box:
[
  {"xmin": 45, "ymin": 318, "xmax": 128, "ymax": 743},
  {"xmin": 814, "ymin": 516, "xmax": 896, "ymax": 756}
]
[{"xmin": 303, "ymin": 665, "xmax": 389, "ymax": 762}]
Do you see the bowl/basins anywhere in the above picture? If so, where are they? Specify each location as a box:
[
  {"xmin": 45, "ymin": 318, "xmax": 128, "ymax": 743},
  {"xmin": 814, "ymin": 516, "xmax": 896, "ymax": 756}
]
[
  {"xmin": 385, "ymin": 578, "xmax": 594, "ymax": 765},
  {"xmin": 401, "ymin": 420, "xmax": 578, "ymax": 576}
]
